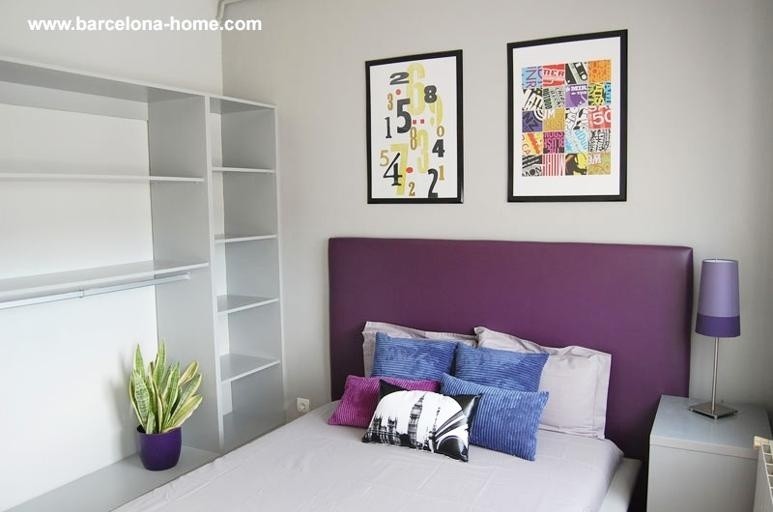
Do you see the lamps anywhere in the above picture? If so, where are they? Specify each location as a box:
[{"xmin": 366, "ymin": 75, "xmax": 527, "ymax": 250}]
[{"xmin": 690, "ymin": 253, "xmax": 742, "ymax": 425}]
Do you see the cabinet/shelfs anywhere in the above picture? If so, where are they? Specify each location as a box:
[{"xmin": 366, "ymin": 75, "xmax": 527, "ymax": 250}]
[
  {"xmin": 0, "ymin": 57, "xmax": 226, "ymax": 511},
  {"xmin": 205, "ymin": 91, "xmax": 292, "ymax": 455}
]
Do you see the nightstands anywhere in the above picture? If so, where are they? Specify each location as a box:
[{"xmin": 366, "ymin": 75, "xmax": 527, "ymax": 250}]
[{"xmin": 645, "ymin": 391, "xmax": 773, "ymax": 512}]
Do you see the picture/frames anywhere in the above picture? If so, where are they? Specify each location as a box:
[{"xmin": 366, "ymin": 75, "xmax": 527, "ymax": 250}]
[
  {"xmin": 505, "ymin": 28, "xmax": 631, "ymax": 204},
  {"xmin": 363, "ymin": 50, "xmax": 464, "ymax": 207}
]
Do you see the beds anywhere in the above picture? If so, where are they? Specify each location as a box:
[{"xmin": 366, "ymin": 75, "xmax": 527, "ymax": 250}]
[{"xmin": 109, "ymin": 238, "xmax": 692, "ymax": 512}]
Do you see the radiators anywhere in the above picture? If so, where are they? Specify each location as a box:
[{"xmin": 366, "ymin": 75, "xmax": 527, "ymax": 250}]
[{"xmin": 751, "ymin": 436, "xmax": 773, "ymax": 512}]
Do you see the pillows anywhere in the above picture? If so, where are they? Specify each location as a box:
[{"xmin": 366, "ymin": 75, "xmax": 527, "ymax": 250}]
[
  {"xmin": 449, "ymin": 343, "xmax": 550, "ymax": 393},
  {"xmin": 363, "ymin": 378, "xmax": 486, "ymax": 463},
  {"xmin": 327, "ymin": 372, "xmax": 436, "ymax": 430},
  {"xmin": 436, "ymin": 369, "xmax": 549, "ymax": 465},
  {"xmin": 472, "ymin": 325, "xmax": 613, "ymax": 441},
  {"xmin": 369, "ymin": 329, "xmax": 451, "ymax": 384},
  {"xmin": 360, "ymin": 320, "xmax": 478, "ymax": 379}
]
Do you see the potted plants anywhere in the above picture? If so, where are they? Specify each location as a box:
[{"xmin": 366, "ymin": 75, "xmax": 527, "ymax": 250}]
[{"xmin": 128, "ymin": 340, "xmax": 203, "ymax": 472}]
